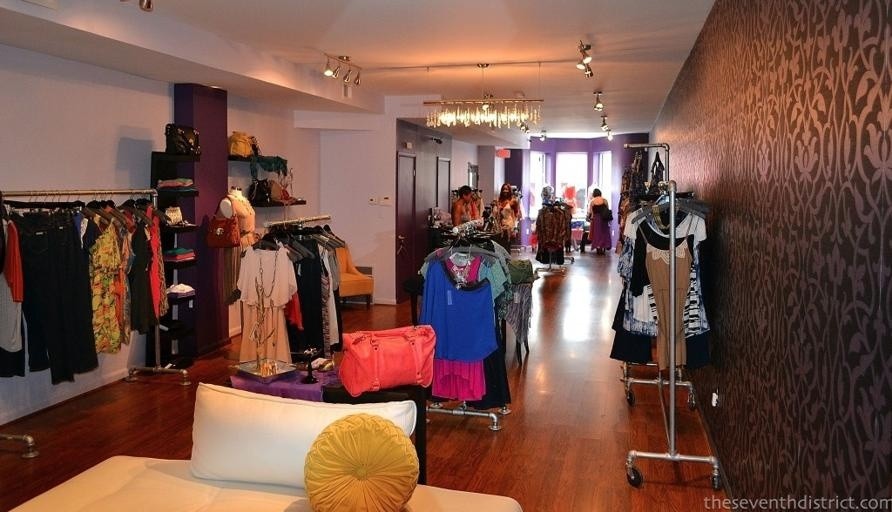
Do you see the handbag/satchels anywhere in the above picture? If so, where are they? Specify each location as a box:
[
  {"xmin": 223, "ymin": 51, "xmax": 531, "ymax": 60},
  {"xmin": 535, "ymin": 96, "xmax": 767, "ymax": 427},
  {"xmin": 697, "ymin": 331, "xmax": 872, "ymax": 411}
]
[
  {"xmin": 165, "ymin": 207, "xmax": 182, "ymax": 226},
  {"xmin": 165, "ymin": 124, "xmax": 200, "ymax": 153},
  {"xmin": 207, "ymin": 196, "xmax": 240, "ymax": 248},
  {"xmin": 601, "ymin": 208, "xmax": 613, "ymax": 221},
  {"xmin": 506, "ymin": 260, "xmax": 533, "ymax": 284},
  {"xmin": 228, "ymin": 131, "xmax": 253, "ymax": 157},
  {"xmin": 249, "ymin": 179, "xmax": 289, "ymax": 201},
  {"xmin": 338, "ymin": 324, "xmax": 436, "ymax": 396}
]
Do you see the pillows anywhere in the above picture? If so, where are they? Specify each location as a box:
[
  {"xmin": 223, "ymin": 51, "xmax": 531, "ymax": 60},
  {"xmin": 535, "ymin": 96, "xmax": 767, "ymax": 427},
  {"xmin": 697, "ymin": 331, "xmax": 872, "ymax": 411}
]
[{"xmin": 192, "ymin": 380, "xmax": 423, "ymax": 512}]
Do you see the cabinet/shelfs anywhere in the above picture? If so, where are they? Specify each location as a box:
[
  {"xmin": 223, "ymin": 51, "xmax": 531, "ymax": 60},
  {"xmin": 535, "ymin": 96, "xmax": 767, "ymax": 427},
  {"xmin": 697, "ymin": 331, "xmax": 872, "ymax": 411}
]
[
  {"xmin": 228, "ymin": 152, "xmax": 307, "ymax": 208},
  {"xmin": 146, "ymin": 151, "xmax": 199, "ymax": 370}
]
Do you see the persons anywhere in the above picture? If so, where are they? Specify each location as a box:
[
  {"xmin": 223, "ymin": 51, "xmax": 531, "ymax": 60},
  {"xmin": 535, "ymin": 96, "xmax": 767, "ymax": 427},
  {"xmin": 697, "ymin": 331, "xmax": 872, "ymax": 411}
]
[
  {"xmin": 588, "ymin": 188, "xmax": 611, "ymax": 255},
  {"xmin": 450, "ymin": 186, "xmax": 472, "ymax": 227},
  {"xmin": 493, "ymin": 184, "xmax": 519, "ymax": 256},
  {"xmin": 219, "ymin": 185, "xmax": 256, "ymax": 303}
]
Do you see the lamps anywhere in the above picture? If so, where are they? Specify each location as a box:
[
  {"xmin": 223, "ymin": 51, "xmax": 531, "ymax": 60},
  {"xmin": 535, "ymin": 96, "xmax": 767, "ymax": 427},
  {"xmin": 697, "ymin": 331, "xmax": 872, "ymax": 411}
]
[
  {"xmin": 421, "ymin": 65, "xmax": 543, "ymax": 129},
  {"xmin": 593, "ymin": 93, "xmax": 604, "ymax": 112},
  {"xmin": 577, "ymin": 51, "xmax": 593, "ymax": 75},
  {"xmin": 601, "ymin": 118, "xmax": 608, "ymax": 132},
  {"xmin": 323, "ymin": 57, "xmax": 361, "ymax": 85}
]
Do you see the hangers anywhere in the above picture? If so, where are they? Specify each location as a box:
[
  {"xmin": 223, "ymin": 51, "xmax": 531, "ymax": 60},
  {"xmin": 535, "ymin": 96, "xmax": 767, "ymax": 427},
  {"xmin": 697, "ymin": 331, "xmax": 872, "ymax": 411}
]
[
  {"xmin": 624, "ymin": 190, "xmax": 713, "ymax": 226},
  {"xmin": 3, "ymin": 189, "xmax": 172, "ymax": 233},
  {"xmin": 236, "ymin": 223, "xmax": 346, "ymax": 266},
  {"xmin": 423, "ymin": 232, "xmax": 497, "ymax": 262}
]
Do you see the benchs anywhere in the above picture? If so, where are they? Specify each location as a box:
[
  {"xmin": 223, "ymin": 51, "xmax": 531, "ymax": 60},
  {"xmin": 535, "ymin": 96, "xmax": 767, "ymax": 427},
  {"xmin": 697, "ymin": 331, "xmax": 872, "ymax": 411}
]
[{"xmin": 8, "ymin": 455, "xmax": 522, "ymax": 512}]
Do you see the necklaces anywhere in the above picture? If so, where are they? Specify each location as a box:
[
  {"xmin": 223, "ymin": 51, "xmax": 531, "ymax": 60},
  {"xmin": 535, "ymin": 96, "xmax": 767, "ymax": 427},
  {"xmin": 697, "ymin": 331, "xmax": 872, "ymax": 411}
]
[
  {"xmin": 227, "ymin": 192, "xmax": 253, "ymax": 214},
  {"xmin": 258, "ymin": 238, "xmax": 278, "ymax": 297},
  {"xmin": 450, "ymin": 243, "xmax": 472, "ymax": 289}
]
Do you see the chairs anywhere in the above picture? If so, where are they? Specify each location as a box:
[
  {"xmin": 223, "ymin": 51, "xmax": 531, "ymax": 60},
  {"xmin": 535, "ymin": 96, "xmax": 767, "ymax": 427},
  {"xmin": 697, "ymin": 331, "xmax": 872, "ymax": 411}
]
[{"xmin": 333, "ymin": 244, "xmax": 375, "ymax": 311}]
